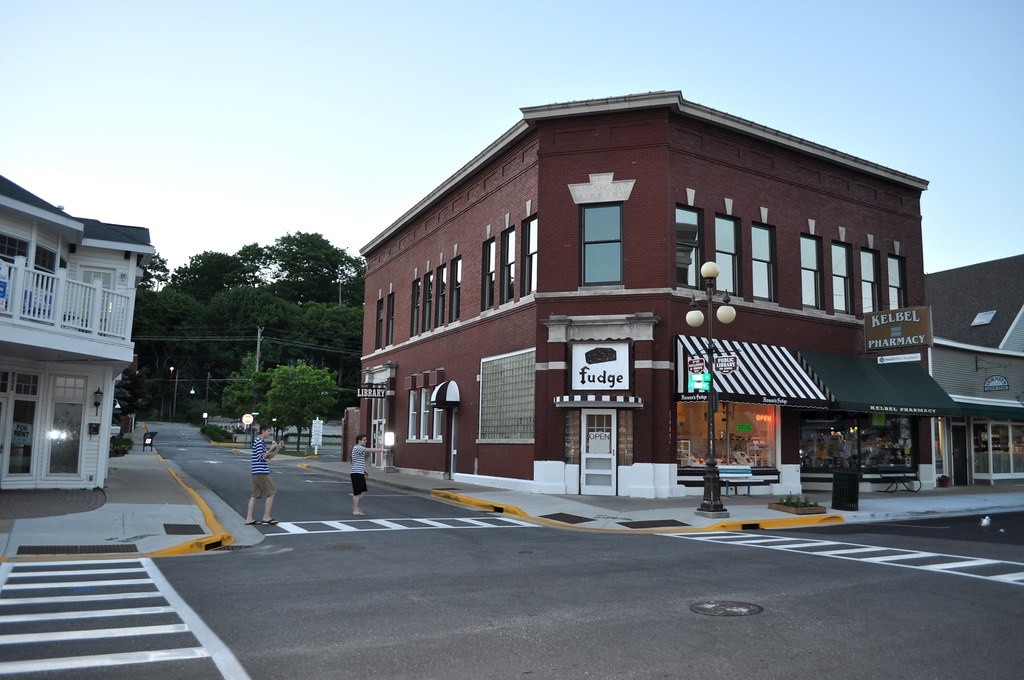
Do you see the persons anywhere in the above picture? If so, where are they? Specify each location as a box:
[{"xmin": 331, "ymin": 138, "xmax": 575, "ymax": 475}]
[
  {"xmin": 245, "ymin": 424, "xmax": 284, "ymax": 525},
  {"xmin": 350, "ymin": 435, "xmax": 390, "ymax": 516}
]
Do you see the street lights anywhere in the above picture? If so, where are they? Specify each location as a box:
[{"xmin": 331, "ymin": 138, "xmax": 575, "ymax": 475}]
[{"xmin": 686, "ymin": 261, "xmax": 735, "ymax": 520}]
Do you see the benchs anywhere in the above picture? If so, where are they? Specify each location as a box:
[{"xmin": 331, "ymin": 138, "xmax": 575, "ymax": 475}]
[
  {"xmin": 715, "ymin": 465, "xmax": 764, "ymax": 496},
  {"xmin": 877, "ymin": 471, "xmax": 922, "ymax": 492}
]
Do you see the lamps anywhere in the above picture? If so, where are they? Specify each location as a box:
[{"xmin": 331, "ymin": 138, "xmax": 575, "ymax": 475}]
[
  {"xmin": 94, "ymin": 387, "xmax": 104, "ymax": 415},
  {"xmin": 113, "ymin": 399, "xmax": 121, "ymax": 408}
]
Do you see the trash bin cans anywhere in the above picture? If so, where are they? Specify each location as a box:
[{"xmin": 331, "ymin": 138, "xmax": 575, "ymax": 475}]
[
  {"xmin": 120, "ymin": 414, "xmax": 136, "ymax": 433},
  {"xmin": 831, "ymin": 467, "xmax": 862, "ymax": 512}
]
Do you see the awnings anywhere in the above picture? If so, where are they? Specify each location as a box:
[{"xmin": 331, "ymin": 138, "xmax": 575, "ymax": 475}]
[
  {"xmin": 799, "ymin": 350, "xmax": 961, "ymax": 416},
  {"xmin": 675, "ymin": 335, "xmax": 830, "ymax": 410},
  {"xmin": 430, "ymin": 381, "xmax": 461, "ymax": 408},
  {"xmin": 956, "ymin": 402, "xmax": 1024, "ymax": 419}
]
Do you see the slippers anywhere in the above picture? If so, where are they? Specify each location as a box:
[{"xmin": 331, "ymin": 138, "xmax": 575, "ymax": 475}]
[
  {"xmin": 245, "ymin": 521, "xmax": 262, "ymax": 525},
  {"xmin": 262, "ymin": 519, "xmax": 278, "ymax": 525}
]
